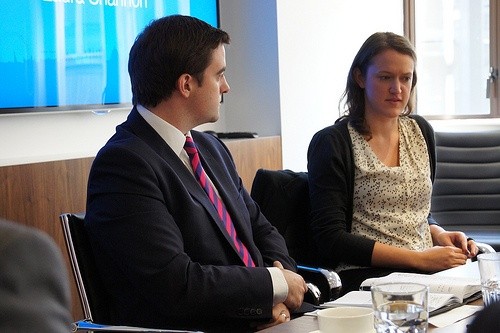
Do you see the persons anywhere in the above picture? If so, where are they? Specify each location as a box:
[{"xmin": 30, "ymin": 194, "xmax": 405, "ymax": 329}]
[
  {"xmin": 86, "ymin": 15, "xmax": 307, "ymax": 332},
  {"xmin": 308, "ymin": 31, "xmax": 480, "ymax": 287}
]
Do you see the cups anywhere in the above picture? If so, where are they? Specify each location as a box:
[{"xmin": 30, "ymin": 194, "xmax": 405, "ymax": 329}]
[
  {"xmin": 370, "ymin": 282, "xmax": 429, "ymax": 333},
  {"xmin": 317, "ymin": 306, "xmax": 375, "ymax": 333},
  {"xmin": 477, "ymin": 252, "xmax": 500, "ymax": 308}
]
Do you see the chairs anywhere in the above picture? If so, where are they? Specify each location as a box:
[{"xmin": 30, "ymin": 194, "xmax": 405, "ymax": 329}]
[
  {"xmin": 256, "ymin": 168, "xmax": 497, "ymax": 301},
  {"xmin": 430, "ymin": 130, "xmax": 500, "ymax": 252},
  {"xmin": 58, "ymin": 212, "xmax": 322, "ymax": 333}
]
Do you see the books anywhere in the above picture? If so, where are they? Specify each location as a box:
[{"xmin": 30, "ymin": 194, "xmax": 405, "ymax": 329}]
[{"xmin": 319, "ymin": 260, "xmax": 481, "ymax": 317}]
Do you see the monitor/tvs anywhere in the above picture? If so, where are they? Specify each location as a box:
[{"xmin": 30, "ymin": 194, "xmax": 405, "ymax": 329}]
[{"xmin": 0, "ymin": 0, "xmax": 222, "ymax": 118}]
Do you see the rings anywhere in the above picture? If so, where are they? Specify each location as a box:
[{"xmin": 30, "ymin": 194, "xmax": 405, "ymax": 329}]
[
  {"xmin": 281, "ymin": 314, "xmax": 286, "ymax": 317},
  {"xmin": 466, "ymin": 237, "xmax": 474, "ymax": 242}
]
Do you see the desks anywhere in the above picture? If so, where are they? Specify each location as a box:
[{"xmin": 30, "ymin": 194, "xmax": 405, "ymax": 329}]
[{"xmin": 256, "ymin": 260, "xmax": 491, "ymax": 333}]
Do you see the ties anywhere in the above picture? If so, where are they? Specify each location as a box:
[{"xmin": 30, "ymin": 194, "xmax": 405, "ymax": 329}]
[{"xmin": 180, "ymin": 133, "xmax": 257, "ymax": 269}]
